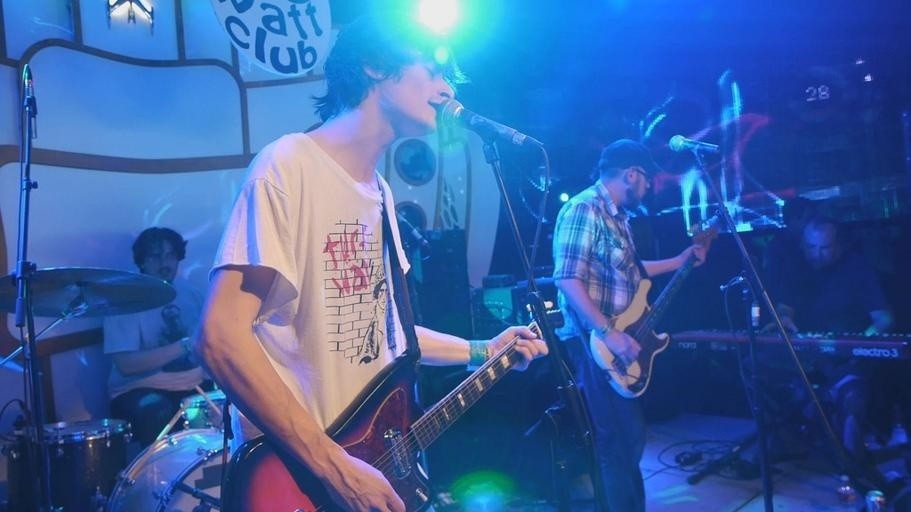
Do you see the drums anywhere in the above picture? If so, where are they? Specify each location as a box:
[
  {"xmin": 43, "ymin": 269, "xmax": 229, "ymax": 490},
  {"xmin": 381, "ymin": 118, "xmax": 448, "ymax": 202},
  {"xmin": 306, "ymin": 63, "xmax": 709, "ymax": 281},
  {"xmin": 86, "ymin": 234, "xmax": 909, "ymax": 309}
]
[
  {"xmin": 181, "ymin": 391, "xmax": 232, "ymax": 431},
  {"xmin": 102, "ymin": 428, "xmax": 231, "ymax": 512},
  {"xmin": 5, "ymin": 416, "xmax": 133, "ymax": 512}
]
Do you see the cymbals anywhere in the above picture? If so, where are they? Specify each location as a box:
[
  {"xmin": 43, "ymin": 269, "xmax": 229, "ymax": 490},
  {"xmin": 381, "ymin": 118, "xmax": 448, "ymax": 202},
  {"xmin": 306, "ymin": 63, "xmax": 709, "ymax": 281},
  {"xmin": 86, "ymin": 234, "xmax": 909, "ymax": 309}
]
[{"xmin": 1, "ymin": 266, "xmax": 178, "ymax": 320}]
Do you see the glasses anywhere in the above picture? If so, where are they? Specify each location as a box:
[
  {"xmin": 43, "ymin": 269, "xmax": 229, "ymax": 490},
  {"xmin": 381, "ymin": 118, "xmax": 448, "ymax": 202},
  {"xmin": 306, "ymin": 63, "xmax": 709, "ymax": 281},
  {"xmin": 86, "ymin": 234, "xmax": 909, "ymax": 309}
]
[{"xmin": 635, "ymin": 168, "xmax": 653, "ymax": 183}]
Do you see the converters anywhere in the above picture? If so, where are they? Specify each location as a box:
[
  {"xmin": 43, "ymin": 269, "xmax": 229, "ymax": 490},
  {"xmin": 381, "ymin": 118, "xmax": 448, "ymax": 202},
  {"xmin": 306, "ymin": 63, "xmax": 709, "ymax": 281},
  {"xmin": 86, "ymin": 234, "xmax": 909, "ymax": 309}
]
[{"xmin": 675, "ymin": 446, "xmax": 703, "ymax": 465}]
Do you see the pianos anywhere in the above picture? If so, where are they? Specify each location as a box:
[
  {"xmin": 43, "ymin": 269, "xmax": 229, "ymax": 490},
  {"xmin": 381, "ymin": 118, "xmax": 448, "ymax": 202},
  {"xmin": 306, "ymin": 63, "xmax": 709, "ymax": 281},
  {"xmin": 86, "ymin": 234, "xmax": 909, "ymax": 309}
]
[{"xmin": 674, "ymin": 329, "xmax": 911, "ymax": 365}]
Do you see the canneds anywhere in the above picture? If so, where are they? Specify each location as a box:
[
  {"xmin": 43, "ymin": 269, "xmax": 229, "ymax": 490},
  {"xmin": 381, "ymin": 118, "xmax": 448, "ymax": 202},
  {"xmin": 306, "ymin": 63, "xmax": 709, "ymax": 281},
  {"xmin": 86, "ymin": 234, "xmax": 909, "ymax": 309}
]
[{"xmin": 865, "ymin": 490, "xmax": 886, "ymax": 512}]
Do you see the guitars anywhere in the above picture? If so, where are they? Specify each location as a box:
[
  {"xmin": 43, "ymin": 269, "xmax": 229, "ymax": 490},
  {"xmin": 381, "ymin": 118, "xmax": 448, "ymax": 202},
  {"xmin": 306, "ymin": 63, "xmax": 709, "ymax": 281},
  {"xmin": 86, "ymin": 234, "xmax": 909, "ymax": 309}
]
[
  {"xmin": 221, "ymin": 293, "xmax": 564, "ymax": 512},
  {"xmin": 589, "ymin": 216, "xmax": 720, "ymax": 400}
]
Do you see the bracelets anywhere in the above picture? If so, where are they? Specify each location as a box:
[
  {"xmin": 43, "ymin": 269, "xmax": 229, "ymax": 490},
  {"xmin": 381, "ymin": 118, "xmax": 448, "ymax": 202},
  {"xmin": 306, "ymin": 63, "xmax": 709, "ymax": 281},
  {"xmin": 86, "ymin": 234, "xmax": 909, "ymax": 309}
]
[
  {"xmin": 596, "ymin": 319, "xmax": 616, "ymax": 343},
  {"xmin": 468, "ymin": 337, "xmax": 489, "ymax": 374}
]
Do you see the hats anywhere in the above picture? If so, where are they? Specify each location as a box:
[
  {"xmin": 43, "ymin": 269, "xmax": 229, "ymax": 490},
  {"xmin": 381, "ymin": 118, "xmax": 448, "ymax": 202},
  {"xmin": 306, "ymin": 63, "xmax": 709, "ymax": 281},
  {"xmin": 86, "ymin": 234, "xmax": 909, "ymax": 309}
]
[{"xmin": 598, "ymin": 138, "xmax": 664, "ymax": 178}]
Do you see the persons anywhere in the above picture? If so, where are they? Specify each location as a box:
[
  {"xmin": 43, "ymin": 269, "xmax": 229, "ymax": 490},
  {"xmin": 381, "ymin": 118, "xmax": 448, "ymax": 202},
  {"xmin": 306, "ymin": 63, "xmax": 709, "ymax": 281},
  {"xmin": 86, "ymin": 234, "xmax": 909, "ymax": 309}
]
[
  {"xmin": 762, "ymin": 196, "xmax": 819, "ymax": 296},
  {"xmin": 553, "ymin": 138, "xmax": 713, "ymax": 512},
  {"xmin": 736, "ymin": 211, "xmax": 898, "ymax": 512},
  {"xmin": 189, "ymin": 7, "xmax": 552, "ymax": 512},
  {"xmin": 101, "ymin": 226, "xmax": 216, "ymax": 451}
]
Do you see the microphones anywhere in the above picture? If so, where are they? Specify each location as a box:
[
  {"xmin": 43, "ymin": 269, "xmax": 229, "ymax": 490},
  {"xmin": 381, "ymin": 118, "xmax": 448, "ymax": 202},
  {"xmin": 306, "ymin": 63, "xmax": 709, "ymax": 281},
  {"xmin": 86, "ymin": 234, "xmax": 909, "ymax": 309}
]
[
  {"xmin": 438, "ymin": 96, "xmax": 543, "ymax": 151},
  {"xmin": 670, "ymin": 133, "xmax": 723, "ymax": 158},
  {"xmin": 750, "ymin": 300, "xmax": 763, "ymax": 336}
]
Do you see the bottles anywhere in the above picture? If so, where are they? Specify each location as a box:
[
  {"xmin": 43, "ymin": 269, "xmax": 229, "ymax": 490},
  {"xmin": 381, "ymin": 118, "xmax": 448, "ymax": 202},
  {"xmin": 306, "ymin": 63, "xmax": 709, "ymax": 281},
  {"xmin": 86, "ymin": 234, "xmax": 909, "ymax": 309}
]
[{"xmin": 836, "ymin": 474, "xmax": 858, "ymax": 512}]
